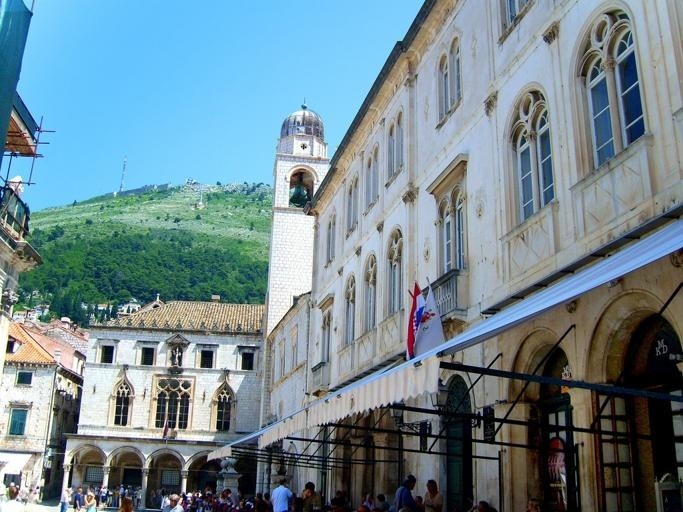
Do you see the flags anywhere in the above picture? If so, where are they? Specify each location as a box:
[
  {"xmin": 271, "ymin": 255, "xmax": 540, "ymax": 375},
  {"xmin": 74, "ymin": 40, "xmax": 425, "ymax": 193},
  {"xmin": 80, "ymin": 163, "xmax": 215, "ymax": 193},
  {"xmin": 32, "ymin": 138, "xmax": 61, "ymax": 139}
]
[
  {"xmin": 405, "ymin": 282, "xmax": 426, "ymax": 360},
  {"xmin": 413, "ymin": 288, "xmax": 446, "ymax": 359}
]
